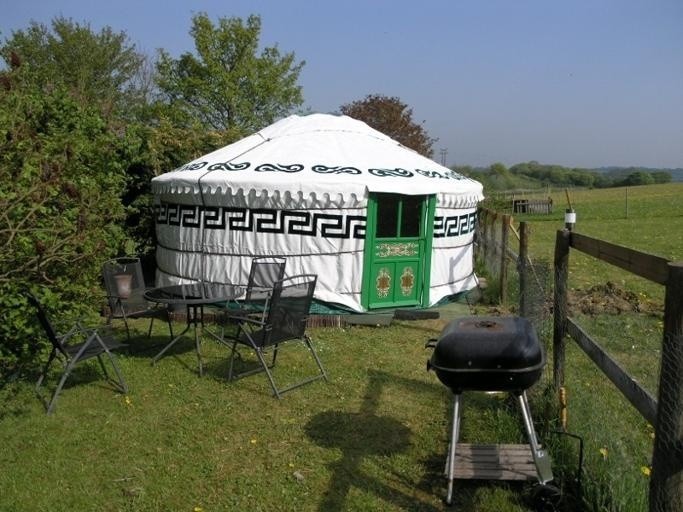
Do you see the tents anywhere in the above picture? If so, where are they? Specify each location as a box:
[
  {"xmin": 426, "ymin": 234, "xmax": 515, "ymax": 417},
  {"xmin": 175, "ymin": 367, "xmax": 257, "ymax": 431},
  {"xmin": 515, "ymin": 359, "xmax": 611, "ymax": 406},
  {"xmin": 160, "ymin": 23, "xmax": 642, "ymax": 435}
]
[{"xmin": 144, "ymin": 109, "xmax": 486, "ymax": 316}]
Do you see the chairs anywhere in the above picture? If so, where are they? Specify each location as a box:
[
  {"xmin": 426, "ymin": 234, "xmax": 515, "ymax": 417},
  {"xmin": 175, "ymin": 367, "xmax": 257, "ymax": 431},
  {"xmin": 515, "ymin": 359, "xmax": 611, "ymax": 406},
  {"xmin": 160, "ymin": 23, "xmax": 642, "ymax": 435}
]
[
  {"xmin": 101, "ymin": 257, "xmax": 174, "ymax": 358},
  {"xmin": 221, "ymin": 256, "xmax": 329, "ymax": 406},
  {"xmin": 21, "ymin": 289, "xmax": 128, "ymax": 414}
]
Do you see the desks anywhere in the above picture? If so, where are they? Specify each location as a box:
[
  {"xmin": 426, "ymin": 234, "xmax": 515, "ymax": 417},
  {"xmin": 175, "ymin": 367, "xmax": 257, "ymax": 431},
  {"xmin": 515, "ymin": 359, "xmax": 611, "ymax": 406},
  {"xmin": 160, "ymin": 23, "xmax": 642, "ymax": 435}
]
[{"xmin": 142, "ymin": 283, "xmax": 245, "ymax": 379}]
